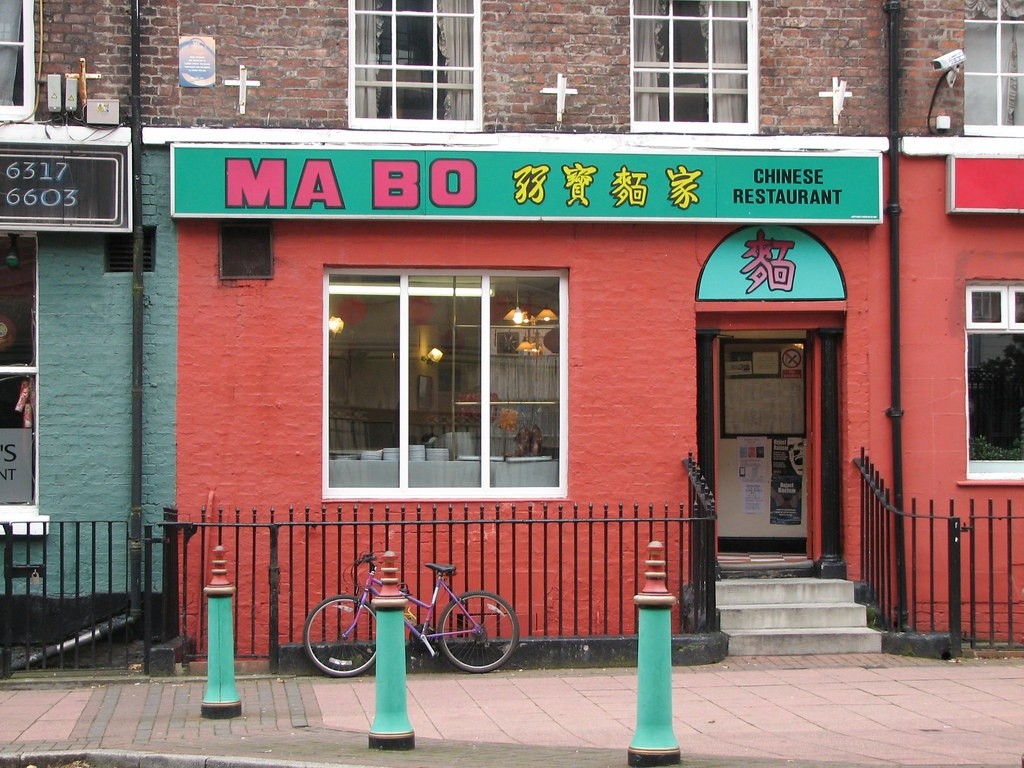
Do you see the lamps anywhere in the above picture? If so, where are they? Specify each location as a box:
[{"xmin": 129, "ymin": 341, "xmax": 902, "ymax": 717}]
[
  {"xmin": 504, "ymin": 279, "xmax": 557, "ymax": 355},
  {"xmin": 329, "ymin": 316, "xmax": 344, "ymax": 338},
  {"xmin": 427, "ymin": 348, "xmax": 443, "ymax": 365}
]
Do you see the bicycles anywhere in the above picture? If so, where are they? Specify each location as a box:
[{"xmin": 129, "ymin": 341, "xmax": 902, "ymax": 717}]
[{"xmin": 302, "ymin": 552, "xmax": 520, "ymax": 678}]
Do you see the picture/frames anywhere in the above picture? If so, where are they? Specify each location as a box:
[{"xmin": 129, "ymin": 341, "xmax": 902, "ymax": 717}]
[{"xmin": 417, "ymin": 375, "xmax": 432, "ymax": 409}]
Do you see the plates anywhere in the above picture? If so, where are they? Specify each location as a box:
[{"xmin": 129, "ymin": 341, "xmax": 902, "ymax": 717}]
[
  {"xmin": 434, "ymin": 432, "xmax": 477, "ymax": 460},
  {"xmin": 409, "ymin": 445, "xmax": 449, "ymax": 461},
  {"xmin": 329, "ymin": 447, "xmax": 400, "ymax": 460}
]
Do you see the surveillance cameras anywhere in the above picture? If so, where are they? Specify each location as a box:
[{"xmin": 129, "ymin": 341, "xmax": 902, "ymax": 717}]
[{"xmin": 931, "ymin": 48, "xmax": 966, "ymax": 72}]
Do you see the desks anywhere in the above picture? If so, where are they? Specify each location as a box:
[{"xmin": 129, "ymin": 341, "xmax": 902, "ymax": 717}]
[{"xmin": 329, "ymin": 455, "xmax": 559, "ymax": 488}]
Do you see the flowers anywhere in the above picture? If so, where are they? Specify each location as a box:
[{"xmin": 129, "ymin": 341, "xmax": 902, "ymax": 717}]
[{"xmin": 457, "ymin": 391, "xmax": 499, "ymax": 423}]
